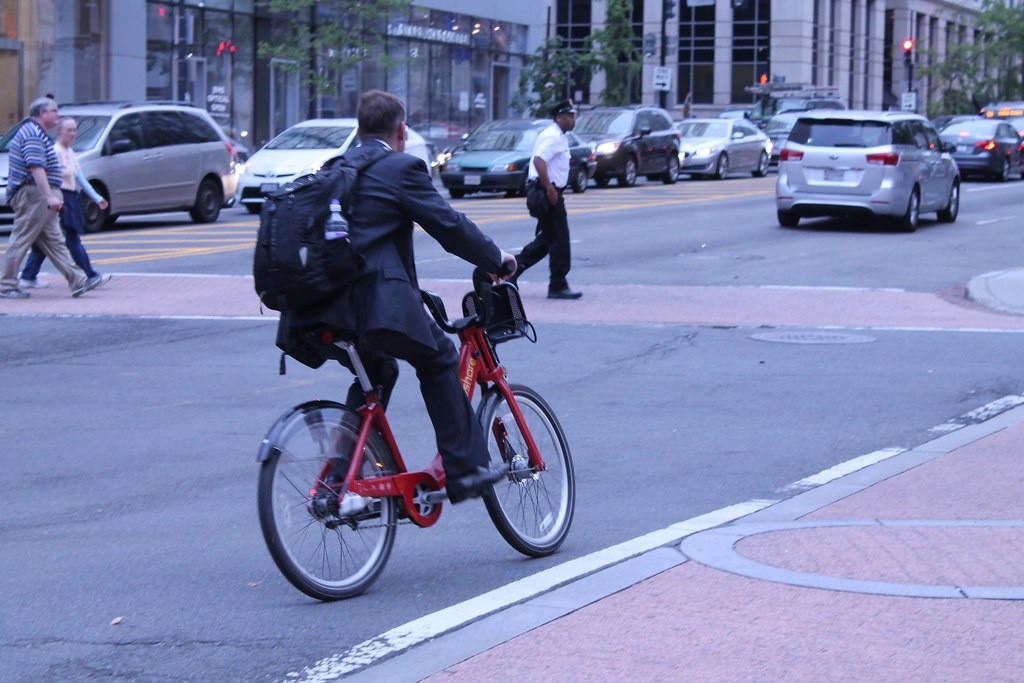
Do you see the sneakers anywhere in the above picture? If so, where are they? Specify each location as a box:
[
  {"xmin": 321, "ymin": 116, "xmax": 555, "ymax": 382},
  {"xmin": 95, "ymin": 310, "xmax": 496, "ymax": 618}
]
[
  {"xmin": 72, "ymin": 275, "xmax": 102, "ymax": 298},
  {"xmin": 88, "ymin": 273, "xmax": 112, "ymax": 290},
  {"xmin": 18, "ymin": 277, "xmax": 49, "ymax": 289},
  {"xmin": 0, "ymin": 289, "xmax": 31, "ymax": 299}
]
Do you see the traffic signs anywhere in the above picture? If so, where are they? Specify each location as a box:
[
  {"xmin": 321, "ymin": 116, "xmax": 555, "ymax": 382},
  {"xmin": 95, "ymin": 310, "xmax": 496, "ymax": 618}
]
[{"xmin": 653, "ymin": 66, "xmax": 672, "ymax": 90}]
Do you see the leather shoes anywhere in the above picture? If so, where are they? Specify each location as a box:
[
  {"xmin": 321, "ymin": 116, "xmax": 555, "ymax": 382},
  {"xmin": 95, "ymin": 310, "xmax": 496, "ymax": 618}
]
[
  {"xmin": 323, "ymin": 471, "xmax": 366, "ymax": 496},
  {"xmin": 444, "ymin": 461, "xmax": 511, "ymax": 505}
]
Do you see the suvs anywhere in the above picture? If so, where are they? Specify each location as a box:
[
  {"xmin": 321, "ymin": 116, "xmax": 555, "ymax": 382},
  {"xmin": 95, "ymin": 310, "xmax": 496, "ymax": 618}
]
[
  {"xmin": 570, "ymin": 104, "xmax": 683, "ymax": 185},
  {"xmin": 0, "ymin": 100, "xmax": 239, "ymax": 234}
]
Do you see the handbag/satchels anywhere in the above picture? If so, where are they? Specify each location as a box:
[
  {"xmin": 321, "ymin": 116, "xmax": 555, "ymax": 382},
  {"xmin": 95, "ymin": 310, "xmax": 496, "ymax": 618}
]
[{"xmin": 526, "ymin": 185, "xmax": 550, "ymax": 218}]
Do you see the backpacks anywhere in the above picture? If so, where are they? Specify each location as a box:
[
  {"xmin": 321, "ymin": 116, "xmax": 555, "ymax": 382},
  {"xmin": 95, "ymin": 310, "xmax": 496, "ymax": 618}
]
[{"xmin": 250, "ymin": 146, "xmax": 399, "ymax": 315}]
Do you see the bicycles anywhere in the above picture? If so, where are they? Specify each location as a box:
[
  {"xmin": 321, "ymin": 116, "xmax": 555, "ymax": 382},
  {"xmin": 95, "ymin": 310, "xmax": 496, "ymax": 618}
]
[{"xmin": 256, "ymin": 257, "xmax": 576, "ymax": 600}]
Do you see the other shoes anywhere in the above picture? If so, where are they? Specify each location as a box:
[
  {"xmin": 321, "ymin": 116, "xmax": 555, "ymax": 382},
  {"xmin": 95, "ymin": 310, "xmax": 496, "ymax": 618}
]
[
  {"xmin": 504, "ymin": 275, "xmax": 519, "ymax": 291},
  {"xmin": 547, "ymin": 287, "xmax": 583, "ymax": 300}
]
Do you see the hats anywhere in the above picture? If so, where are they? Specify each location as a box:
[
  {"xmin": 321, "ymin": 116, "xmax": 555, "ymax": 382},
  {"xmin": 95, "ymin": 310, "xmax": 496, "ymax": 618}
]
[{"xmin": 549, "ymin": 98, "xmax": 579, "ymax": 116}]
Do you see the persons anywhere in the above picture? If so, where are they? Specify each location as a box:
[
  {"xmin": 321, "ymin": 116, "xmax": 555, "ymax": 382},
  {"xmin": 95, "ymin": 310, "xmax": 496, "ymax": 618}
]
[
  {"xmin": 506, "ymin": 98, "xmax": 583, "ymax": 299},
  {"xmin": 0, "ymin": 97, "xmax": 103, "ymax": 300},
  {"xmin": 18, "ymin": 116, "xmax": 112, "ymax": 289},
  {"xmin": 275, "ymin": 90, "xmax": 519, "ymax": 506}
]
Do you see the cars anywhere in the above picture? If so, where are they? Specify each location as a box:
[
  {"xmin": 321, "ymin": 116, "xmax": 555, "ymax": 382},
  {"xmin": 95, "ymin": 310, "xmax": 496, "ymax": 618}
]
[
  {"xmin": 933, "ymin": 100, "xmax": 1024, "ymax": 181},
  {"xmin": 412, "ymin": 122, "xmax": 472, "ymax": 159},
  {"xmin": 744, "ymin": 95, "xmax": 960, "ymax": 231},
  {"xmin": 673, "ymin": 117, "xmax": 773, "ymax": 181},
  {"xmin": 438, "ymin": 118, "xmax": 598, "ymax": 197},
  {"xmin": 235, "ymin": 118, "xmax": 432, "ymax": 213}
]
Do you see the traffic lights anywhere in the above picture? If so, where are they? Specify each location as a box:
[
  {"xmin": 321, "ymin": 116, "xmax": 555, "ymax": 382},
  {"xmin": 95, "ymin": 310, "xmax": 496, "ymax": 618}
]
[
  {"xmin": 904, "ymin": 40, "xmax": 912, "ymax": 66},
  {"xmin": 758, "ymin": 73, "xmax": 768, "ymax": 87},
  {"xmin": 665, "ymin": 0, "xmax": 677, "ymax": 19}
]
